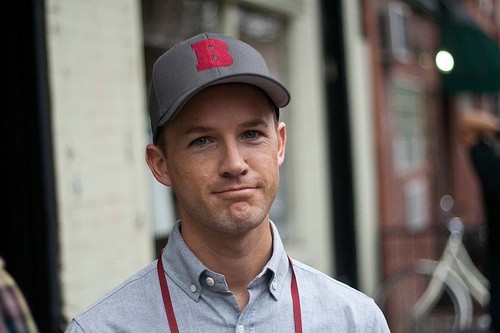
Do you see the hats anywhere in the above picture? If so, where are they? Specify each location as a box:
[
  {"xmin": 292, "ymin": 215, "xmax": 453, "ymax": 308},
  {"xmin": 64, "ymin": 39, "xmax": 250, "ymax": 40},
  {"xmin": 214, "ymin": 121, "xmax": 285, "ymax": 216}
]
[{"xmin": 148, "ymin": 32, "xmax": 291, "ymax": 132}]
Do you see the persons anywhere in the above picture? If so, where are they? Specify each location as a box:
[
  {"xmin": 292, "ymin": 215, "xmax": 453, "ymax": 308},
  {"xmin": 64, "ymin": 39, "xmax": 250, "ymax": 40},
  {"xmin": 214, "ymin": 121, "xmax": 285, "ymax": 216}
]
[
  {"xmin": 63, "ymin": 33, "xmax": 391, "ymax": 333},
  {"xmin": 433, "ymin": 0, "xmax": 500, "ymax": 333}
]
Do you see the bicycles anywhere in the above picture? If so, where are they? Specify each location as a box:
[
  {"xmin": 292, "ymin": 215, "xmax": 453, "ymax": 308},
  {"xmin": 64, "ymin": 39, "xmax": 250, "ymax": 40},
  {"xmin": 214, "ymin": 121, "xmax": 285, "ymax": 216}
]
[{"xmin": 369, "ymin": 179, "xmax": 500, "ymax": 332}]
